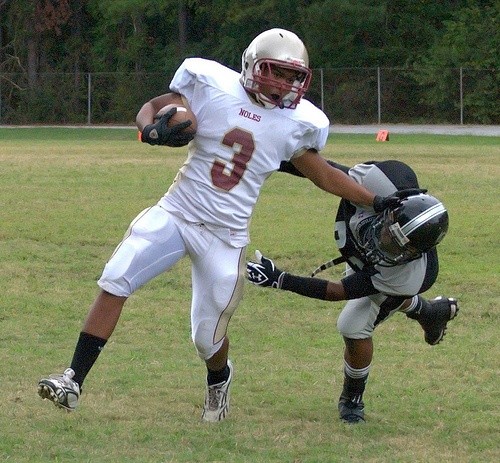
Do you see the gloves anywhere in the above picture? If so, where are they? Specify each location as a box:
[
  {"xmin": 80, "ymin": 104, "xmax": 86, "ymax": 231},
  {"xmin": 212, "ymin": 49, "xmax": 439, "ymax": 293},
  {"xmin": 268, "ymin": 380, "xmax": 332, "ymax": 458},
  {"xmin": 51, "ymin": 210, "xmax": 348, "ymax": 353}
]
[
  {"xmin": 141, "ymin": 107, "xmax": 196, "ymax": 147},
  {"xmin": 373, "ymin": 188, "xmax": 428, "ymax": 213},
  {"xmin": 246, "ymin": 250, "xmax": 285, "ymax": 288}
]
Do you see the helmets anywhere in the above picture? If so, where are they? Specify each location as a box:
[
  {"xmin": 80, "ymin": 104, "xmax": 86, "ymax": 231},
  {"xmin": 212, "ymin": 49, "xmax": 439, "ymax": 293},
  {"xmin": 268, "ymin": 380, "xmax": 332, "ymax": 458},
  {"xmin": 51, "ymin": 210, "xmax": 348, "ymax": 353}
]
[
  {"xmin": 385, "ymin": 193, "xmax": 449, "ymax": 252},
  {"xmin": 242, "ymin": 27, "xmax": 309, "ymax": 109}
]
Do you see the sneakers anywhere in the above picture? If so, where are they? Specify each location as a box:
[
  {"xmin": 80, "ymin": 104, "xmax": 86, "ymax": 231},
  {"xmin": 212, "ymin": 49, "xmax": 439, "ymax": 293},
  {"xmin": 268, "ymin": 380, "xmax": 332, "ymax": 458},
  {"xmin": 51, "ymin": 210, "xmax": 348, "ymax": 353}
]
[
  {"xmin": 37, "ymin": 368, "xmax": 80, "ymax": 413},
  {"xmin": 425, "ymin": 296, "xmax": 459, "ymax": 346},
  {"xmin": 338, "ymin": 388, "xmax": 366, "ymax": 425},
  {"xmin": 201, "ymin": 359, "xmax": 233, "ymax": 423}
]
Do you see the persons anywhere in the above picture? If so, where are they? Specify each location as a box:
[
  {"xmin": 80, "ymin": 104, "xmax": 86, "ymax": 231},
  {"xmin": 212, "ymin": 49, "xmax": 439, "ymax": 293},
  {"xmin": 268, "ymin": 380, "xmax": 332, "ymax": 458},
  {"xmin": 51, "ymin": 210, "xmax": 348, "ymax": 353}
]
[
  {"xmin": 246, "ymin": 156, "xmax": 460, "ymax": 422},
  {"xmin": 37, "ymin": 29, "xmax": 429, "ymax": 424}
]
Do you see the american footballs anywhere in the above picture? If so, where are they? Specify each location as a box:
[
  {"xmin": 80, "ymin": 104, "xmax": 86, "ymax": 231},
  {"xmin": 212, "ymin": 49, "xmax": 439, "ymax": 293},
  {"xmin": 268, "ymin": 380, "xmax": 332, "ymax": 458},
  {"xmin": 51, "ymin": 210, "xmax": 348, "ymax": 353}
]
[{"xmin": 153, "ymin": 104, "xmax": 197, "ymax": 135}]
